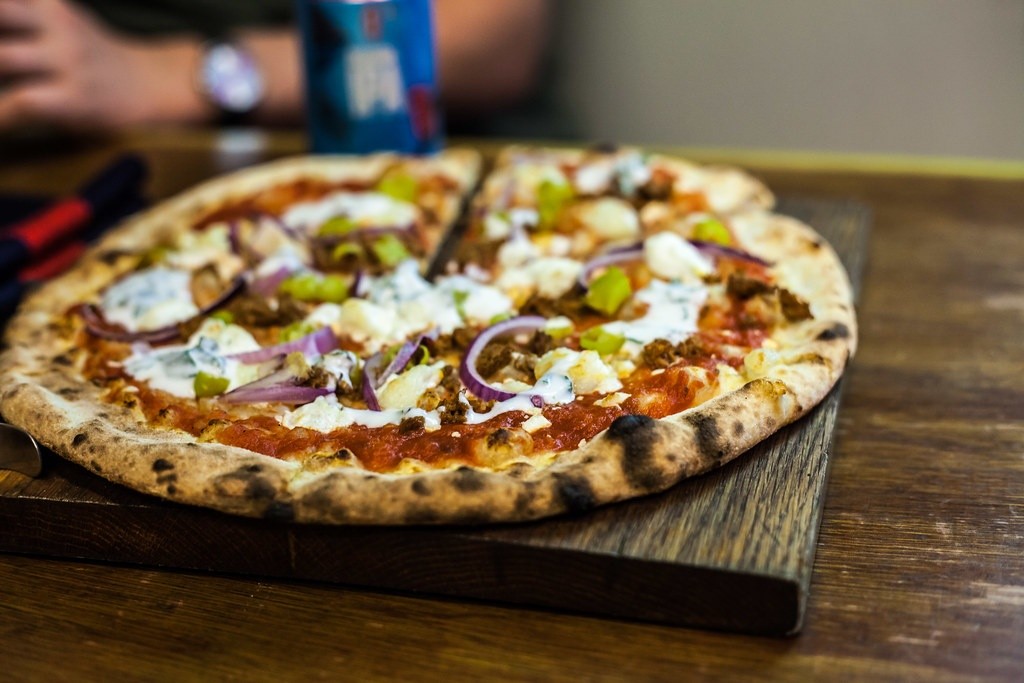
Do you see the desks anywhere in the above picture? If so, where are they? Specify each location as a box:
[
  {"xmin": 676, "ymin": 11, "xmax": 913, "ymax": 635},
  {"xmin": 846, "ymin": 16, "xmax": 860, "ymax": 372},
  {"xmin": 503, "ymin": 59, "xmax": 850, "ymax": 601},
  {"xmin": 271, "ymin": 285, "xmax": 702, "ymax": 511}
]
[{"xmin": 0, "ymin": 138, "xmax": 1024, "ymax": 683}]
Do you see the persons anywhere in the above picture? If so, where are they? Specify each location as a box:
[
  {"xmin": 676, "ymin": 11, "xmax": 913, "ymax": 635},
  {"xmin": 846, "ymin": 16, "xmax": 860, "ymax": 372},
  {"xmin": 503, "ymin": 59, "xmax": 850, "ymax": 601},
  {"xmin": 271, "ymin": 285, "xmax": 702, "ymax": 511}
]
[{"xmin": 0, "ymin": 0, "xmax": 548, "ymax": 136}]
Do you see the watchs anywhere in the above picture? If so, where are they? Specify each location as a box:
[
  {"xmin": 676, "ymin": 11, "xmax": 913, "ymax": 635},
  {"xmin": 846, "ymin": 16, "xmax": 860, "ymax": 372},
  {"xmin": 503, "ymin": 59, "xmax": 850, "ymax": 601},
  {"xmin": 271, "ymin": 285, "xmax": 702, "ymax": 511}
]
[{"xmin": 197, "ymin": 26, "xmax": 262, "ymax": 125}]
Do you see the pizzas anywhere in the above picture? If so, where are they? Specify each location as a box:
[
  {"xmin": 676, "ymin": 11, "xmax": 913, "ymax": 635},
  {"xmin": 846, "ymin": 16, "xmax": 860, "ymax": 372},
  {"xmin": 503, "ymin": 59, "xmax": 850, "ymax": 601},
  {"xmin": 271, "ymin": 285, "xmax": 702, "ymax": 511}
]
[{"xmin": 0, "ymin": 144, "xmax": 858, "ymax": 529}]
